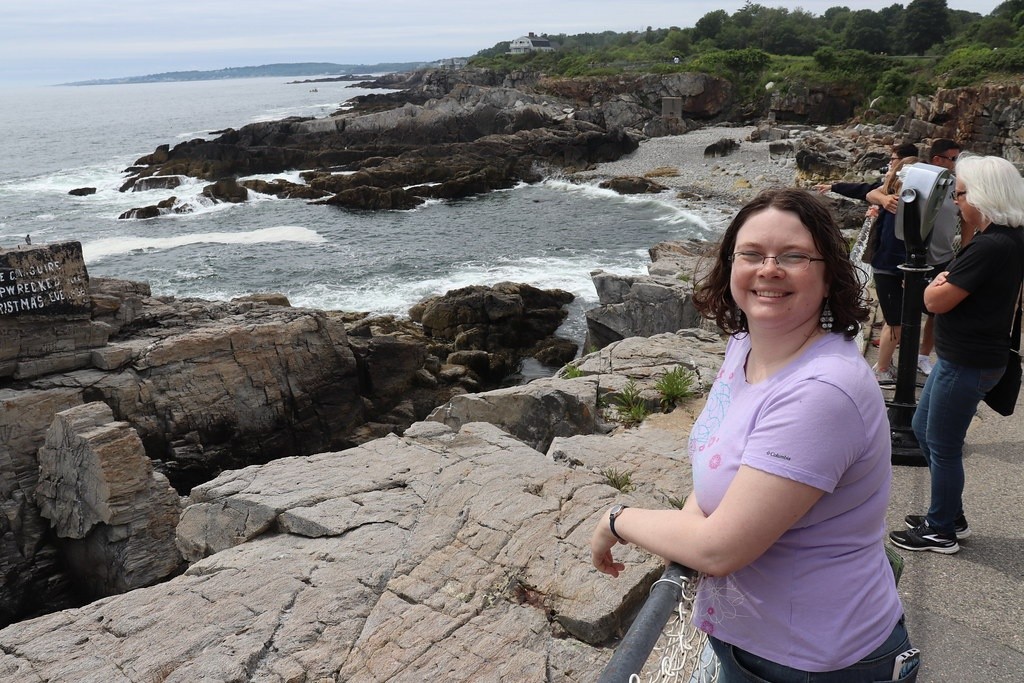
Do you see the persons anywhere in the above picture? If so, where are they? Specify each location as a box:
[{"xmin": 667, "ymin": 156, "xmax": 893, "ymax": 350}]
[
  {"xmin": 862, "ymin": 138, "xmax": 975, "ymax": 390},
  {"xmin": 888, "ymin": 154, "xmax": 1023, "ymax": 554},
  {"xmin": 590, "ymin": 186, "xmax": 924, "ymax": 683}
]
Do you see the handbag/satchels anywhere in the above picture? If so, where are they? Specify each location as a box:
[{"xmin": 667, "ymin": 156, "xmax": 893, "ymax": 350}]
[
  {"xmin": 983, "ymin": 355, "xmax": 1022, "ymax": 416},
  {"xmin": 862, "ymin": 216, "xmax": 883, "ymax": 262}
]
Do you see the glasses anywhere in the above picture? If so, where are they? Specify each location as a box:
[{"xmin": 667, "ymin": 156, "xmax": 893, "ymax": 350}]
[
  {"xmin": 891, "ymin": 157, "xmax": 898, "ymax": 160},
  {"xmin": 728, "ymin": 250, "xmax": 824, "ymax": 271},
  {"xmin": 939, "ymin": 155, "xmax": 958, "ymax": 161},
  {"xmin": 951, "ymin": 190, "xmax": 966, "ymax": 200}
]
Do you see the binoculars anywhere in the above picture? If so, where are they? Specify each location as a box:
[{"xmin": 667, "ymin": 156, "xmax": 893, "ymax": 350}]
[{"xmin": 893, "ymin": 161, "xmax": 954, "ymax": 240}]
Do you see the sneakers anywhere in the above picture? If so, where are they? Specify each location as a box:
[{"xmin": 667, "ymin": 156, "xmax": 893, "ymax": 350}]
[
  {"xmin": 889, "ymin": 520, "xmax": 960, "ymax": 554},
  {"xmin": 905, "ymin": 515, "xmax": 972, "ymax": 539}
]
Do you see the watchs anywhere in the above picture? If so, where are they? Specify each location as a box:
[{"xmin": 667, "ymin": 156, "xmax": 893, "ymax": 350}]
[{"xmin": 609, "ymin": 504, "xmax": 632, "ymax": 546}]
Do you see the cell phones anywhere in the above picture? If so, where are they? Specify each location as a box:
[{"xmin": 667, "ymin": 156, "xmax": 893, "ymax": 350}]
[{"xmin": 892, "ymin": 648, "xmax": 920, "ymax": 681}]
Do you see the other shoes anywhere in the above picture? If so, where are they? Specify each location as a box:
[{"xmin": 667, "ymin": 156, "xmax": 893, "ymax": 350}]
[
  {"xmin": 872, "ymin": 368, "xmax": 894, "ymax": 385},
  {"xmin": 917, "ymin": 355, "xmax": 932, "ymax": 377},
  {"xmin": 889, "ymin": 359, "xmax": 898, "ymax": 377}
]
